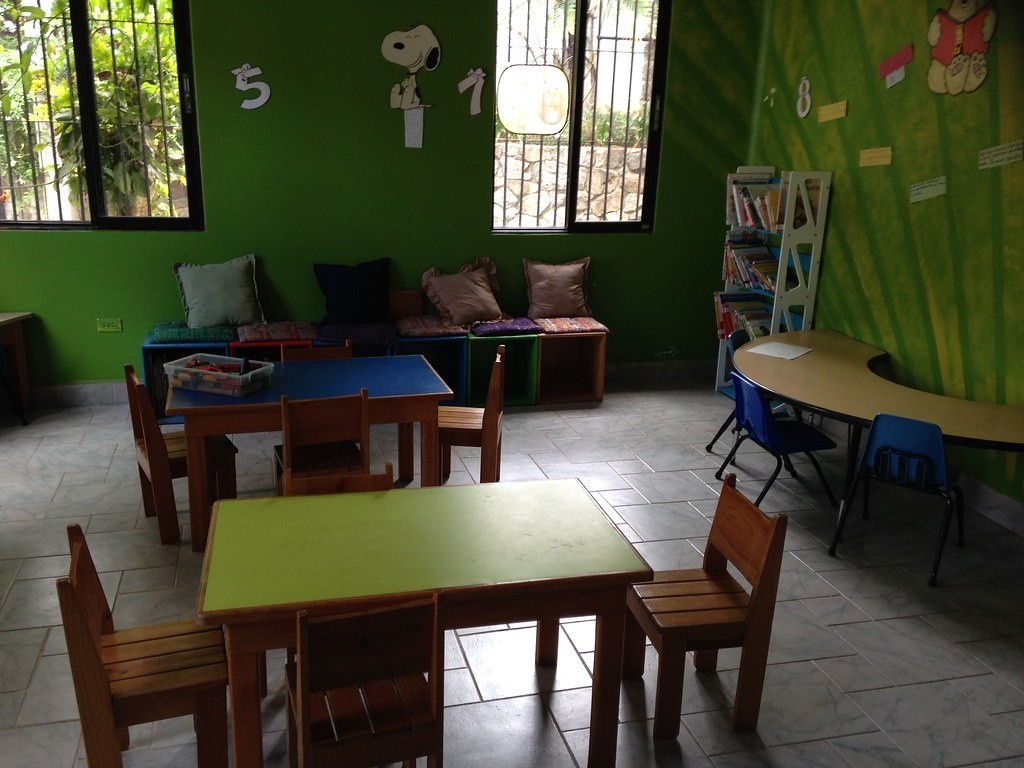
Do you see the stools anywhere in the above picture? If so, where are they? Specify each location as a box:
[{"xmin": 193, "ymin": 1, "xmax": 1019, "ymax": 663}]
[{"xmin": 142, "ymin": 318, "xmax": 609, "ymax": 423}]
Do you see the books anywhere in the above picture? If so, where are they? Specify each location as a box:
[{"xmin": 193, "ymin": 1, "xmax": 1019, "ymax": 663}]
[{"xmin": 714, "ymin": 170, "xmax": 821, "ymax": 341}]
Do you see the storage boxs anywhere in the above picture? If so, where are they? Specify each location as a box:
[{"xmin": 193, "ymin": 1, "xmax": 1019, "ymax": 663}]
[{"xmin": 164, "ymin": 353, "xmax": 275, "ymax": 396}]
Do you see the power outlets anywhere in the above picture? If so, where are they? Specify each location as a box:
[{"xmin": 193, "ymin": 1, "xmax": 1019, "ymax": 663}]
[{"xmin": 97, "ymin": 318, "xmax": 123, "ymax": 333}]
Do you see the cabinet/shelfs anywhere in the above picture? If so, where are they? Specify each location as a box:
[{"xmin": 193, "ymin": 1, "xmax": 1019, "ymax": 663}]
[{"xmin": 714, "ymin": 165, "xmax": 832, "ymax": 416}]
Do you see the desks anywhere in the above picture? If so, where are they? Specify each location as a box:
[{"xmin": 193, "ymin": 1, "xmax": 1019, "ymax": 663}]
[
  {"xmin": 165, "ymin": 353, "xmax": 455, "ymax": 554},
  {"xmin": 733, "ymin": 326, "xmax": 1024, "ymax": 500},
  {"xmin": 197, "ymin": 479, "xmax": 654, "ymax": 768},
  {"xmin": 0, "ymin": 311, "xmax": 35, "ymax": 409}
]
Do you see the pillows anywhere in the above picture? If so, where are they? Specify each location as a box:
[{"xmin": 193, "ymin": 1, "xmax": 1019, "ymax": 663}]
[
  {"xmin": 523, "ymin": 256, "xmax": 594, "ymax": 318},
  {"xmin": 317, "ymin": 256, "xmax": 395, "ymax": 329},
  {"xmin": 423, "ymin": 258, "xmax": 512, "ymax": 329},
  {"xmin": 173, "ymin": 253, "xmax": 266, "ymax": 330}
]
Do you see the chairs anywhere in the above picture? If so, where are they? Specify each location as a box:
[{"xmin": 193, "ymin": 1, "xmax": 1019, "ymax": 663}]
[
  {"xmin": 56, "ymin": 521, "xmax": 229, "ymax": 768},
  {"xmin": 271, "ymin": 340, "xmax": 394, "ymax": 496},
  {"xmin": 621, "ymin": 474, "xmax": 787, "ymax": 739},
  {"xmin": 286, "ymin": 592, "xmax": 448, "ymax": 768},
  {"xmin": 437, "ymin": 343, "xmax": 514, "ymax": 481},
  {"xmin": 706, "ymin": 328, "xmax": 838, "ymax": 509},
  {"xmin": 124, "ymin": 363, "xmax": 240, "ymax": 542},
  {"xmin": 829, "ymin": 411, "xmax": 970, "ymax": 587}
]
